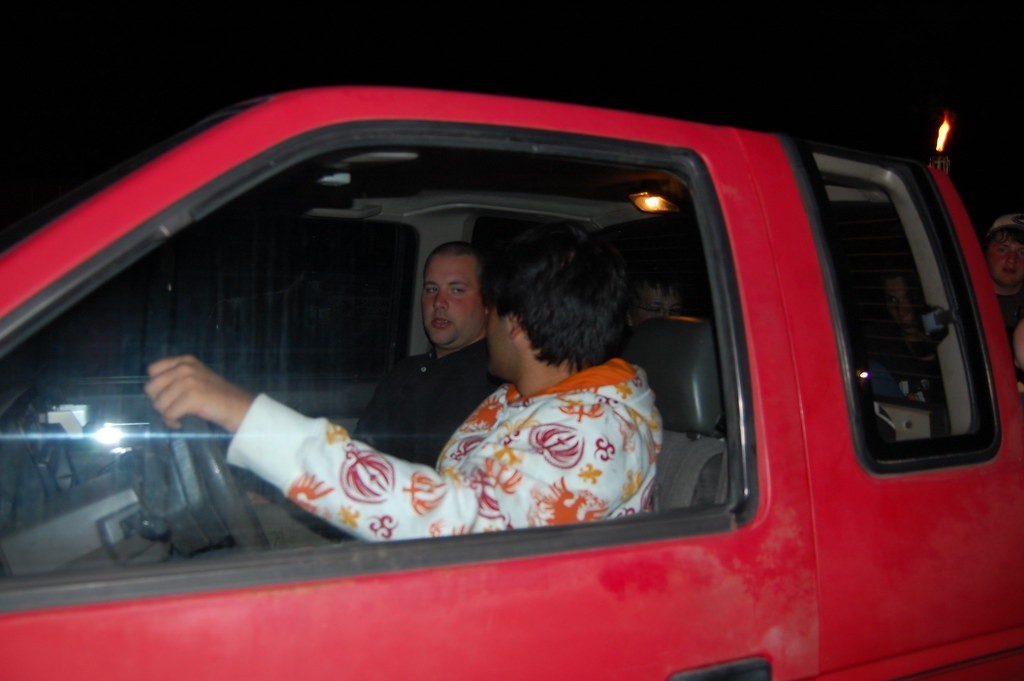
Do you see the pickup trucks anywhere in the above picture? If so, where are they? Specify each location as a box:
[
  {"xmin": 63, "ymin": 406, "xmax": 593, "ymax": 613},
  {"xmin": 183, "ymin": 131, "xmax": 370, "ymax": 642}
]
[{"xmin": 2, "ymin": 83, "xmax": 1024, "ymax": 678}]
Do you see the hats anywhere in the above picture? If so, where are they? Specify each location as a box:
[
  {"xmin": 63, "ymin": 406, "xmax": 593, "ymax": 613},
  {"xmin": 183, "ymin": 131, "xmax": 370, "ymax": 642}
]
[{"xmin": 986, "ymin": 214, "xmax": 1024, "ymax": 237}]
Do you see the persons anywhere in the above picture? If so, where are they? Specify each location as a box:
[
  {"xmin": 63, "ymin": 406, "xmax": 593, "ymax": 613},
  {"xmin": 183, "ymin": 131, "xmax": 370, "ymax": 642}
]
[
  {"xmin": 141, "ymin": 215, "xmax": 665, "ymax": 541},
  {"xmin": 626, "ymin": 273, "xmax": 682, "ymax": 326},
  {"xmin": 984, "ymin": 212, "xmax": 1024, "ymax": 384},
  {"xmin": 197, "ymin": 240, "xmax": 507, "ymax": 558},
  {"xmin": 873, "ymin": 265, "xmax": 943, "ymax": 395}
]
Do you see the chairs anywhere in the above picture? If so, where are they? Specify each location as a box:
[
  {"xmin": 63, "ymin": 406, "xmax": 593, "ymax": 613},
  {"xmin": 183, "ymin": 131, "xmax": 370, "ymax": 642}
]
[{"xmin": 615, "ymin": 320, "xmax": 729, "ymax": 514}]
